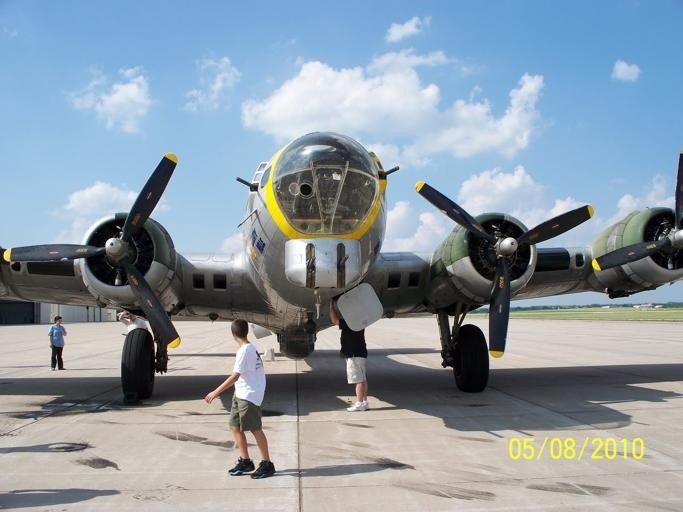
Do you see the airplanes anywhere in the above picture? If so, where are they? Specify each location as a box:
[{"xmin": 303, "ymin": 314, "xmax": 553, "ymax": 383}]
[{"xmin": 0, "ymin": 132, "xmax": 683, "ymax": 401}]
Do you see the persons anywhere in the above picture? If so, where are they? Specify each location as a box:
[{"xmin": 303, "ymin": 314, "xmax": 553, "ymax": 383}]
[
  {"xmin": 328, "ymin": 297, "xmax": 370, "ymax": 411},
  {"xmin": 47, "ymin": 316, "xmax": 67, "ymax": 370},
  {"xmin": 204, "ymin": 319, "xmax": 275, "ymax": 478},
  {"xmin": 118, "ymin": 309, "xmax": 148, "ymax": 337}
]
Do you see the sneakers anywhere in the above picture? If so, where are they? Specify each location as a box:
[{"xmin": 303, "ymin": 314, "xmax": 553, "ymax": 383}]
[
  {"xmin": 250, "ymin": 461, "xmax": 275, "ymax": 479},
  {"xmin": 347, "ymin": 400, "xmax": 371, "ymax": 411},
  {"xmin": 227, "ymin": 457, "xmax": 255, "ymax": 475},
  {"xmin": 51, "ymin": 367, "xmax": 67, "ymax": 371}
]
